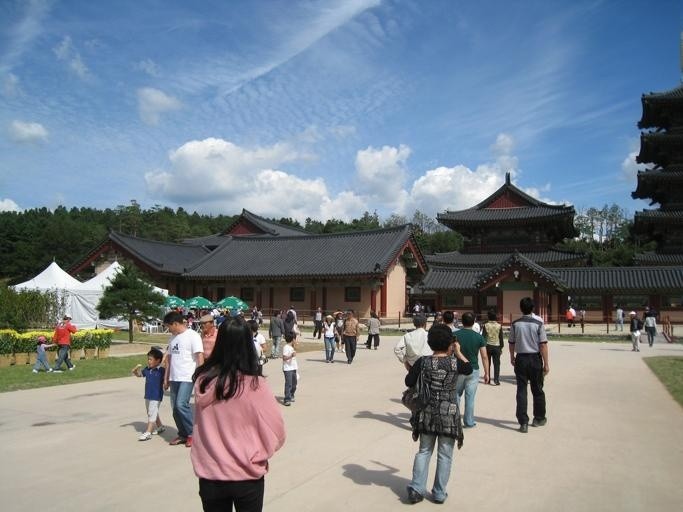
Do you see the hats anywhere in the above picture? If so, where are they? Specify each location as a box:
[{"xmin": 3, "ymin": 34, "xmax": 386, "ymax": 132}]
[
  {"xmin": 38, "ymin": 335, "xmax": 47, "ymax": 343},
  {"xmin": 628, "ymin": 311, "xmax": 637, "ymax": 315},
  {"xmin": 65, "ymin": 314, "xmax": 73, "ymax": 319},
  {"xmin": 324, "ymin": 316, "xmax": 337, "ymax": 322},
  {"xmin": 198, "ymin": 315, "xmax": 214, "ymax": 322}
]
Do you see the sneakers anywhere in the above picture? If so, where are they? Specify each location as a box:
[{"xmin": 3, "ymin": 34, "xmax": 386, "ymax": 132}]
[
  {"xmin": 182, "ymin": 437, "xmax": 193, "ymax": 445},
  {"xmin": 348, "ymin": 358, "xmax": 353, "ymax": 364},
  {"xmin": 69, "ymin": 365, "xmax": 75, "ymax": 369},
  {"xmin": 434, "ymin": 492, "xmax": 449, "ymax": 503},
  {"xmin": 33, "ymin": 369, "xmax": 37, "ymax": 372},
  {"xmin": 54, "ymin": 369, "xmax": 62, "ymax": 373},
  {"xmin": 327, "ymin": 359, "xmax": 335, "ymax": 363},
  {"xmin": 292, "ymin": 395, "xmax": 295, "ymax": 401},
  {"xmin": 171, "ymin": 435, "xmax": 186, "ymax": 447},
  {"xmin": 285, "ymin": 398, "xmax": 291, "ymax": 406},
  {"xmin": 139, "ymin": 432, "xmax": 151, "ymax": 441},
  {"xmin": 46, "ymin": 368, "xmax": 52, "ymax": 372},
  {"xmin": 154, "ymin": 424, "xmax": 165, "ymax": 435}
]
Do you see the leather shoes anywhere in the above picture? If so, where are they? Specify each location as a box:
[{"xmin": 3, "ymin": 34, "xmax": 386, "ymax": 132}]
[
  {"xmin": 533, "ymin": 418, "xmax": 547, "ymax": 427},
  {"xmin": 410, "ymin": 485, "xmax": 425, "ymax": 503},
  {"xmin": 495, "ymin": 378, "xmax": 501, "ymax": 384},
  {"xmin": 520, "ymin": 423, "xmax": 529, "ymax": 431},
  {"xmin": 486, "ymin": 380, "xmax": 490, "ymax": 382}
]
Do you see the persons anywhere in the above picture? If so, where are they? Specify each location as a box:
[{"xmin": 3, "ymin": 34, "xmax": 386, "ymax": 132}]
[
  {"xmin": 247, "ymin": 320, "xmax": 267, "ymax": 377},
  {"xmin": 333, "ymin": 310, "xmax": 347, "ymax": 353},
  {"xmin": 269, "ymin": 311, "xmax": 285, "ymax": 359},
  {"xmin": 287, "ymin": 305, "xmax": 297, "ymax": 334},
  {"xmin": 132, "ymin": 349, "xmax": 169, "ymax": 442},
  {"xmin": 482, "ymin": 311, "xmax": 504, "ymax": 385},
  {"xmin": 33, "ymin": 335, "xmax": 57, "ymax": 373},
  {"xmin": 177, "ymin": 307, "xmax": 214, "ymax": 329},
  {"xmin": 643, "ymin": 306, "xmax": 657, "ymax": 347},
  {"xmin": 283, "ymin": 331, "xmax": 298, "ymax": 406},
  {"xmin": 629, "ymin": 311, "xmax": 643, "ymax": 352},
  {"xmin": 413, "ymin": 302, "xmax": 424, "ymax": 314},
  {"xmin": 443, "ymin": 311, "xmax": 460, "ymax": 333},
  {"xmin": 413, "ymin": 303, "xmax": 419, "ymax": 313},
  {"xmin": 433, "ymin": 312, "xmax": 443, "ymax": 325},
  {"xmin": 313, "ymin": 307, "xmax": 324, "ymax": 339},
  {"xmin": 472, "ymin": 315, "xmax": 481, "ymax": 333},
  {"xmin": 199, "ymin": 315, "xmax": 218, "ymax": 363},
  {"xmin": 53, "ymin": 315, "xmax": 77, "ymax": 373},
  {"xmin": 343, "ymin": 308, "xmax": 360, "ymax": 364},
  {"xmin": 394, "ymin": 313, "xmax": 435, "ymax": 371},
  {"xmin": 642, "ymin": 307, "xmax": 651, "ymax": 320},
  {"xmin": 323, "ymin": 315, "xmax": 337, "ymax": 363},
  {"xmin": 250, "ymin": 306, "xmax": 264, "ymax": 327},
  {"xmin": 508, "ymin": 296, "xmax": 549, "ymax": 433},
  {"xmin": 190, "ymin": 316, "xmax": 286, "ymax": 512},
  {"xmin": 566, "ymin": 307, "xmax": 574, "ymax": 328},
  {"xmin": 531, "ymin": 299, "xmax": 544, "ymax": 323},
  {"xmin": 366, "ymin": 313, "xmax": 380, "ymax": 350},
  {"xmin": 284, "ymin": 311, "xmax": 298, "ymax": 348},
  {"xmin": 164, "ymin": 311, "xmax": 204, "ymax": 448},
  {"xmin": 569, "ymin": 306, "xmax": 576, "ymax": 327},
  {"xmin": 364, "ymin": 311, "xmax": 375, "ymax": 345},
  {"xmin": 615, "ymin": 305, "xmax": 624, "ymax": 332},
  {"xmin": 213, "ymin": 308, "xmax": 244, "ymax": 325},
  {"xmin": 453, "ymin": 312, "xmax": 490, "ymax": 428},
  {"xmin": 405, "ymin": 324, "xmax": 470, "ymax": 504}
]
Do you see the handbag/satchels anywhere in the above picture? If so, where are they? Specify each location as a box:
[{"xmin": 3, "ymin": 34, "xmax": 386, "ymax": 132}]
[{"xmin": 402, "ymin": 356, "xmax": 429, "ymax": 411}]
